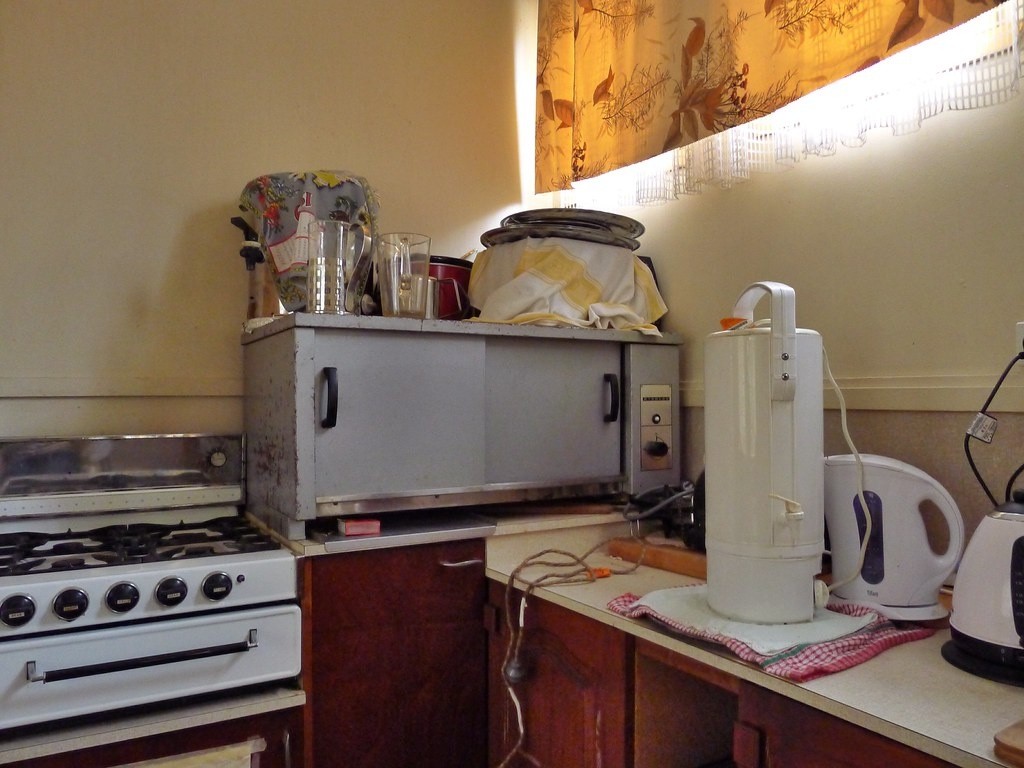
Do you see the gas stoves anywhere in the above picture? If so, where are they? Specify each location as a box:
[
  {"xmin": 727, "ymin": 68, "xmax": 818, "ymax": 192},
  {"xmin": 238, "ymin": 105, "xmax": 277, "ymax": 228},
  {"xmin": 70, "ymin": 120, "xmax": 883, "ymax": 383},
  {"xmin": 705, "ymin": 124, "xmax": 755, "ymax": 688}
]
[{"xmin": 0, "ymin": 433, "xmax": 298, "ymax": 640}]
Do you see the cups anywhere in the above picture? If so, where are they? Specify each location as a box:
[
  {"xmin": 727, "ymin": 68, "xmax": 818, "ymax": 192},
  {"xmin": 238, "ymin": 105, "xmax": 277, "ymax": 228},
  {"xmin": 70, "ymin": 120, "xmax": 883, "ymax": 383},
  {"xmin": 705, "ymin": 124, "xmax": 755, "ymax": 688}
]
[
  {"xmin": 376, "ymin": 232, "xmax": 431, "ymax": 318},
  {"xmin": 307, "ymin": 220, "xmax": 348, "ymax": 314}
]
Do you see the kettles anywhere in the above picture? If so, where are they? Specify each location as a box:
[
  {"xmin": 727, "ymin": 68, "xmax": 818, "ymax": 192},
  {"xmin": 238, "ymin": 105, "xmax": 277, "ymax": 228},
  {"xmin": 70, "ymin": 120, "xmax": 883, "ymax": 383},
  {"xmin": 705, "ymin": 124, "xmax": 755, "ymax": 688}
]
[
  {"xmin": 822, "ymin": 452, "xmax": 965, "ymax": 630},
  {"xmin": 941, "ymin": 488, "xmax": 1024, "ymax": 688}
]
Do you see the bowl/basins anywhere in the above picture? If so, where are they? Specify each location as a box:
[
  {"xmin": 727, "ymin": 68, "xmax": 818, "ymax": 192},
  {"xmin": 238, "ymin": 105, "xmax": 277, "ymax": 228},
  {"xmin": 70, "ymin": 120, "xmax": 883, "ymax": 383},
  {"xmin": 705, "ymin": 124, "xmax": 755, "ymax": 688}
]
[{"xmin": 387, "ymin": 254, "xmax": 474, "ymax": 319}]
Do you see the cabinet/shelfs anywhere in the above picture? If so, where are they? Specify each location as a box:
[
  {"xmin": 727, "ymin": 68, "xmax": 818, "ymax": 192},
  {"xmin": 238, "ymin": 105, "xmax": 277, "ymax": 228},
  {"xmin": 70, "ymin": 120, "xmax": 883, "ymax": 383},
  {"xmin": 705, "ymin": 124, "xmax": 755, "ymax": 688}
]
[
  {"xmin": 261, "ymin": 520, "xmax": 496, "ymax": 768},
  {"xmin": 488, "ymin": 566, "xmax": 1023, "ymax": 767}
]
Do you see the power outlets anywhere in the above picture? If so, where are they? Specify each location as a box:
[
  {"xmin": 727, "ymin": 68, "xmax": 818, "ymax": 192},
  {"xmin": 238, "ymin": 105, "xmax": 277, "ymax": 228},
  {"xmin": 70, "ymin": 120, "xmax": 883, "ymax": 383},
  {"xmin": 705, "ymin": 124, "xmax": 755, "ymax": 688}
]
[{"xmin": 1015, "ymin": 323, "xmax": 1024, "ymax": 363}]
